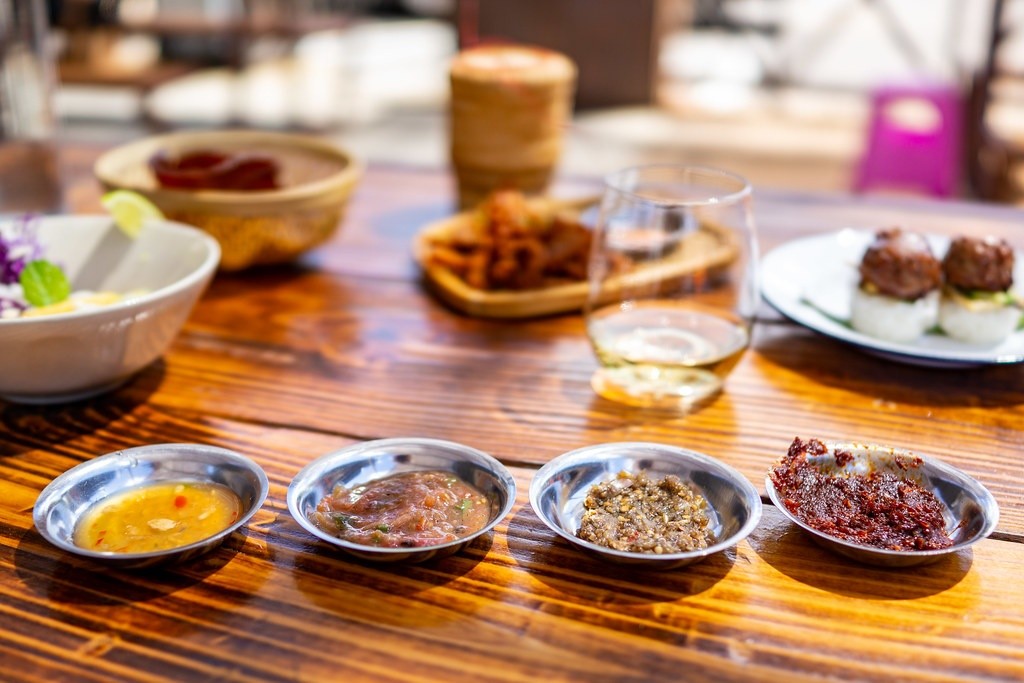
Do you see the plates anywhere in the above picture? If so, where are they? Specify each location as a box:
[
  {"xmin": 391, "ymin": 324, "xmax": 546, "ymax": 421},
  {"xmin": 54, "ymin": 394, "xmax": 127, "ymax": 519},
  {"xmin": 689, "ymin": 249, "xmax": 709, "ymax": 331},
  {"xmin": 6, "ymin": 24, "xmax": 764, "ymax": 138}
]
[
  {"xmin": 766, "ymin": 447, "xmax": 1000, "ymax": 566},
  {"xmin": 286, "ymin": 438, "xmax": 516, "ymax": 561},
  {"xmin": 32, "ymin": 443, "xmax": 268, "ymax": 566},
  {"xmin": 759, "ymin": 230, "xmax": 1024, "ymax": 370},
  {"xmin": 410, "ymin": 194, "xmax": 744, "ymax": 317},
  {"xmin": 528, "ymin": 443, "xmax": 763, "ymax": 568}
]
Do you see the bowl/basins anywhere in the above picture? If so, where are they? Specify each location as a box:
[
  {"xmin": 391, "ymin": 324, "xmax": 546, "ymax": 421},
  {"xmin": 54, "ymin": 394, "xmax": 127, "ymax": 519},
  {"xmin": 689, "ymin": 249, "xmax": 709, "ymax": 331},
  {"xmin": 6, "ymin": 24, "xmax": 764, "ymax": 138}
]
[
  {"xmin": 0, "ymin": 216, "xmax": 221, "ymax": 403},
  {"xmin": 92, "ymin": 131, "xmax": 360, "ymax": 271}
]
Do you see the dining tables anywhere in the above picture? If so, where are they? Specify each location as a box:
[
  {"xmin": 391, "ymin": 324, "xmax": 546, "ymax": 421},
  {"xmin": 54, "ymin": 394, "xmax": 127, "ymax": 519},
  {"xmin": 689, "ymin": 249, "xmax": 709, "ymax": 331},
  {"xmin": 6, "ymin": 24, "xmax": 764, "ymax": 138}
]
[{"xmin": 1, "ymin": 140, "xmax": 1024, "ymax": 683}]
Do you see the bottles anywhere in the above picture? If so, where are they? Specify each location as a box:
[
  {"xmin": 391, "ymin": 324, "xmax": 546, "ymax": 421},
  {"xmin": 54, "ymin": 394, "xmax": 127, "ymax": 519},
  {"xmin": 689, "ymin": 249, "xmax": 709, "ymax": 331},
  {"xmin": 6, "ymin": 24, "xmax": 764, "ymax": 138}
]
[
  {"xmin": 2, "ymin": 0, "xmax": 58, "ymax": 212},
  {"xmin": 449, "ymin": 44, "xmax": 575, "ymax": 196}
]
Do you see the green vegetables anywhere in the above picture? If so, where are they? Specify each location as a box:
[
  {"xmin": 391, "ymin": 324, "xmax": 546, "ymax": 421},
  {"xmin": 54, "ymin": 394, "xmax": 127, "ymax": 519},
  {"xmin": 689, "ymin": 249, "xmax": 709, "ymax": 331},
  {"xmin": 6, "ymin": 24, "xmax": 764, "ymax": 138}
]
[{"xmin": 20, "ymin": 257, "xmax": 70, "ymax": 304}]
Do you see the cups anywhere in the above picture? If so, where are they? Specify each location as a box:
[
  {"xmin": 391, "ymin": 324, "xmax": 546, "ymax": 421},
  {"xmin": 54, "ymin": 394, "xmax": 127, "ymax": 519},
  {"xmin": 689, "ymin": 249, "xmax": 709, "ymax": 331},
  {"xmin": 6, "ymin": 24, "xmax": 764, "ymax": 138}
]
[{"xmin": 585, "ymin": 165, "xmax": 752, "ymax": 403}]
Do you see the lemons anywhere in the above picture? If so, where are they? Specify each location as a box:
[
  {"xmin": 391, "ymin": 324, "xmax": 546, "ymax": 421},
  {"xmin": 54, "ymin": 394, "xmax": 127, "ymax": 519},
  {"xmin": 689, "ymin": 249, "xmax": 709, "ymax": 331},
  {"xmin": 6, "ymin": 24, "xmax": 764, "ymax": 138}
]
[{"xmin": 98, "ymin": 190, "xmax": 165, "ymax": 239}]
